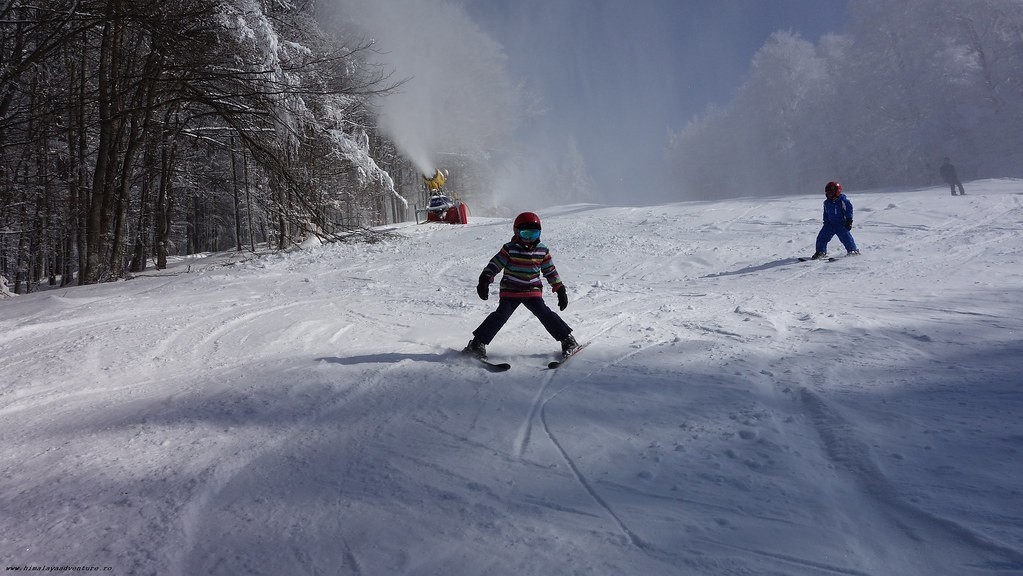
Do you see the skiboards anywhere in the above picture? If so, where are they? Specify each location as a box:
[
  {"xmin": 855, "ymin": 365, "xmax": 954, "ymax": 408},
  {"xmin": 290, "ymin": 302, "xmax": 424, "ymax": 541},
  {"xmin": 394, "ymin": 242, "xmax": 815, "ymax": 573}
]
[
  {"xmin": 548, "ymin": 341, "xmax": 594, "ymax": 369},
  {"xmin": 462, "ymin": 347, "xmax": 510, "ymax": 372},
  {"xmin": 797, "ymin": 252, "xmax": 856, "ymax": 262}
]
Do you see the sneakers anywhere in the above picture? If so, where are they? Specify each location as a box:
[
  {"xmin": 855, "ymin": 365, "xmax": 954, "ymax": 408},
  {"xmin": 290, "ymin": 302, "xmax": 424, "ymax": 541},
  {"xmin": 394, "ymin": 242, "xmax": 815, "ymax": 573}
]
[
  {"xmin": 845, "ymin": 249, "xmax": 859, "ymax": 256},
  {"xmin": 561, "ymin": 334, "xmax": 578, "ymax": 359},
  {"xmin": 811, "ymin": 251, "xmax": 826, "ymax": 260},
  {"xmin": 465, "ymin": 340, "xmax": 488, "ymax": 361}
]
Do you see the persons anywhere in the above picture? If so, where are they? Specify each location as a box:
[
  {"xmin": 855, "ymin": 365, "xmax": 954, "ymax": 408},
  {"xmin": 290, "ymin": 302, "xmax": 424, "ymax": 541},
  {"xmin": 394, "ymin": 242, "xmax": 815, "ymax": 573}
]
[
  {"xmin": 812, "ymin": 182, "xmax": 859, "ymax": 259},
  {"xmin": 465, "ymin": 212, "xmax": 579, "ymax": 360},
  {"xmin": 940, "ymin": 157, "xmax": 964, "ymax": 196}
]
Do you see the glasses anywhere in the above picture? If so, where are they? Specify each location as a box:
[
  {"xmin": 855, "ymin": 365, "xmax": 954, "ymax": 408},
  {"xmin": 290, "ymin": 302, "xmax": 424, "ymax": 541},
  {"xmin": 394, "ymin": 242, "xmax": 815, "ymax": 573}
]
[
  {"xmin": 519, "ymin": 228, "xmax": 540, "ymax": 240},
  {"xmin": 825, "ymin": 186, "xmax": 836, "ymax": 192}
]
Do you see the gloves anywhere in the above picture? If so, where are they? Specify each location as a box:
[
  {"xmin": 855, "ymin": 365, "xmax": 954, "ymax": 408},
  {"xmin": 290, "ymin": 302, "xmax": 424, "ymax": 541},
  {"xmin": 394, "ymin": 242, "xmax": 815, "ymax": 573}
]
[
  {"xmin": 557, "ymin": 286, "xmax": 567, "ymax": 311},
  {"xmin": 846, "ymin": 218, "xmax": 852, "ymax": 231},
  {"xmin": 477, "ymin": 279, "xmax": 489, "ymax": 300}
]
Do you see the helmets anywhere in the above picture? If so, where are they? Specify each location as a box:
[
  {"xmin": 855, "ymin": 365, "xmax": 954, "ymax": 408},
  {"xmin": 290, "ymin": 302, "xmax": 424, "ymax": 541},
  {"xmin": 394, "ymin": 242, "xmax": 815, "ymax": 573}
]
[
  {"xmin": 513, "ymin": 212, "xmax": 542, "ymax": 240},
  {"xmin": 826, "ymin": 182, "xmax": 842, "ymax": 196}
]
